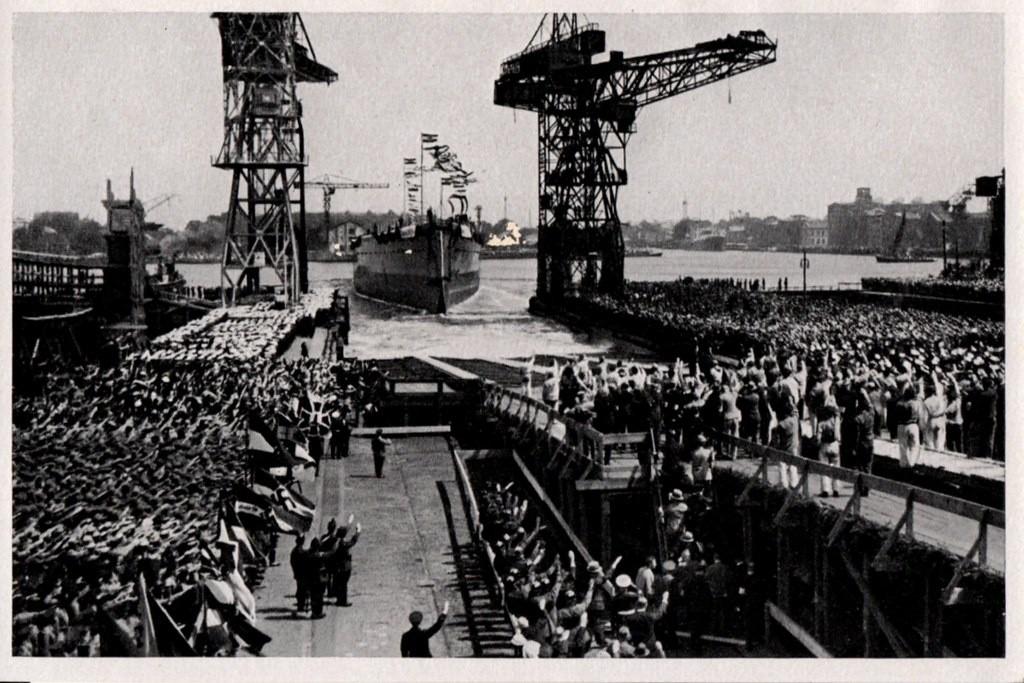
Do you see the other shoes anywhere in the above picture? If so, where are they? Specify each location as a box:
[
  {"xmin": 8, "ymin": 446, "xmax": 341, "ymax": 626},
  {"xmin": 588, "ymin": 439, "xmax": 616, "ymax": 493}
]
[
  {"xmin": 335, "ymin": 602, "xmax": 353, "ymax": 607},
  {"xmin": 310, "ymin": 613, "xmax": 325, "ymax": 619}
]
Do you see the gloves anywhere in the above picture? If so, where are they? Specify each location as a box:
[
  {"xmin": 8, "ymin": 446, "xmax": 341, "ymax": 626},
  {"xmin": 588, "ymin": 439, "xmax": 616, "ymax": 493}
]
[
  {"xmin": 347, "ymin": 515, "xmax": 353, "ymax": 525},
  {"xmin": 356, "ymin": 522, "xmax": 360, "ymax": 532},
  {"xmin": 441, "ymin": 601, "xmax": 450, "ymax": 614}
]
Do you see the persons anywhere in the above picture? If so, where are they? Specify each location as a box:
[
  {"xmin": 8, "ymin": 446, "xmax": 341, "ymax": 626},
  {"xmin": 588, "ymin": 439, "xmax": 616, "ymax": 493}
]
[
  {"xmin": 12, "ymin": 293, "xmax": 392, "ymax": 658},
  {"xmin": 481, "ymin": 277, "xmax": 1006, "ymax": 657},
  {"xmin": 400, "ymin": 601, "xmax": 449, "ymax": 658}
]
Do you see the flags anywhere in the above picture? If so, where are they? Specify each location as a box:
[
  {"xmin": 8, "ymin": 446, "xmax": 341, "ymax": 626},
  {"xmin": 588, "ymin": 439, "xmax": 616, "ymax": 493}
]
[{"xmin": 404, "ymin": 133, "xmax": 474, "ymax": 212}]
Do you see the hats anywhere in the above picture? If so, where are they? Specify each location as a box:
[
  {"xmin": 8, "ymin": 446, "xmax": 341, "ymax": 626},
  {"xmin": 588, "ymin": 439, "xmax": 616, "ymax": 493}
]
[
  {"xmin": 552, "ymin": 627, "xmax": 569, "ymax": 643},
  {"xmin": 409, "ymin": 611, "xmax": 422, "ymax": 625},
  {"xmin": 587, "ymin": 561, "xmax": 602, "ymax": 573},
  {"xmin": 615, "ymin": 574, "xmax": 632, "ymax": 587},
  {"xmin": 615, "ymin": 626, "xmax": 632, "ymax": 640},
  {"xmin": 669, "ymin": 489, "xmax": 684, "ymax": 500},
  {"xmin": 679, "ymin": 532, "xmax": 694, "ymax": 542},
  {"xmin": 633, "ymin": 643, "xmax": 650, "ymax": 657},
  {"xmin": 661, "ymin": 561, "xmax": 677, "ymax": 573}
]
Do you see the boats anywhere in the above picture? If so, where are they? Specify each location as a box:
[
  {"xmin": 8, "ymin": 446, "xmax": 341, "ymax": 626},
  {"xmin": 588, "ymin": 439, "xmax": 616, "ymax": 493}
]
[{"xmin": 353, "ymin": 133, "xmax": 480, "ymax": 317}]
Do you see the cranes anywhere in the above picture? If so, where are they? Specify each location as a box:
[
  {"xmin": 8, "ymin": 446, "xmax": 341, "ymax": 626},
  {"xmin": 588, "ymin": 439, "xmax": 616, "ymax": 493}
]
[
  {"xmin": 493, "ymin": 14, "xmax": 776, "ymax": 304},
  {"xmin": 211, "ymin": 12, "xmax": 339, "ymax": 321},
  {"xmin": 291, "ymin": 181, "xmax": 390, "ymax": 256}
]
